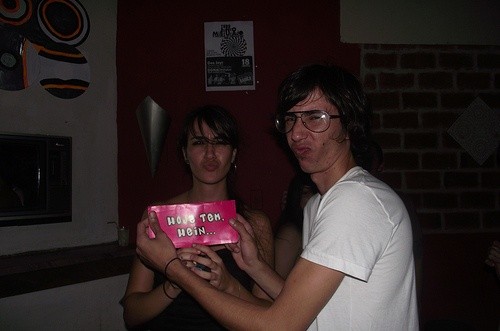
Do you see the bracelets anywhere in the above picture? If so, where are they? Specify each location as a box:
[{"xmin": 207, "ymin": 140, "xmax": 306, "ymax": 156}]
[
  {"xmin": 164, "ymin": 257, "xmax": 180, "ymax": 279},
  {"xmin": 238, "ymin": 280, "xmax": 241, "ymax": 298},
  {"xmin": 163, "ymin": 276, "xmax": 177, "ymax": 300}
]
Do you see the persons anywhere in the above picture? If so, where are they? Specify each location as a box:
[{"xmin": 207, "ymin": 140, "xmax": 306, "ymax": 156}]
[
  {"xmin": 136, "ymin": 61, "xmax": 418, "ymax": 331},
  {"xmin": 274, "ymin": 174, "xmax": 319, "ymax": 280},
  {"xmin": 123, "ymin": 103, "xmax": 274, "ymax": 331}
]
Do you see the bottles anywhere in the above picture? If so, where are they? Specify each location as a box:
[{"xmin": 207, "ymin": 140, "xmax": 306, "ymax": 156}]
[{"xmin": 118, "ymin": 224, "xmax": 128, "ymax": 246}]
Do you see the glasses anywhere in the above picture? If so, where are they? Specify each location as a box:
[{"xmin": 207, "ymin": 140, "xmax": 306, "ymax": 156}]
[{"xmin": 273, "ymin": 110, "xmax": 341, "ymax": 134}]
